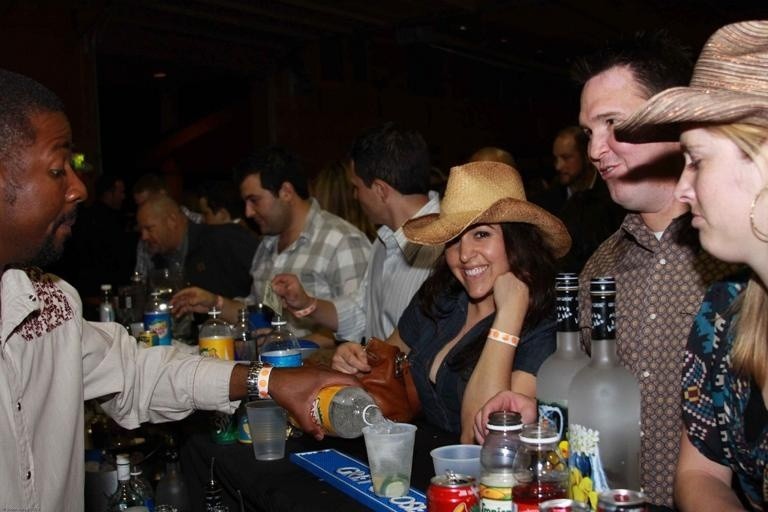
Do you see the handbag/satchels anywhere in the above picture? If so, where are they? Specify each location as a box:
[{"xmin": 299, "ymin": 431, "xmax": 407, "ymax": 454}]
[{"xmin": 355, "ymin": 338, "xmax": 423, "ymax": 423}]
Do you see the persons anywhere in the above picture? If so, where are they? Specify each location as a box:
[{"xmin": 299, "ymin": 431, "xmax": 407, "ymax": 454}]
[
  {"xmin": 614, "ymin": 20, "xmax": 768, "ymax": 512},
  {"xmin": 49, "ymin": 119, "xmax": 623, "ymax": 446},
  {"xmin": 0, "ymin": 67, "xmax": 366, "ymax": 512},
  {"xmin": 473, "ymin": 29, "xmax": 747, "ymax": 512}
]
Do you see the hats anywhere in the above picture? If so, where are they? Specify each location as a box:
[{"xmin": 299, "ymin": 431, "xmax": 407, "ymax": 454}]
[
  {"xmin": 614, "ymin": 20, "xmax": 768, "ymax": 143},
  {"xmin": 404, "ymin": 161, "xmax": 572, "ymax": 259}
]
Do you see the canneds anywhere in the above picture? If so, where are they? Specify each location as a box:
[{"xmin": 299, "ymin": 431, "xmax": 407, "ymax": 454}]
[
  {"xmin": 597, "ymin": 489, "xmax": 649, "ymax": 512},
  {"xmin": 427, "ymin": 469, "xmax": 480, "ymax": 512},
  {"xmin": 139, "ymin": 331, "xmax": 159, "ymax": 347},
  {"xmin": 208, "ymin": 408, "xmax": 239, "ymax": 443},
  {"xmin": 540, "ymin": 499, "xmax": 573, "ymax": 512}
]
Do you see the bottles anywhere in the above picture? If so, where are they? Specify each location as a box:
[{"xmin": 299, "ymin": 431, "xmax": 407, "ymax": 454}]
[
  {"xmin": 197, "ymin": 304, "xmax": 235, "ymax": 362},
  {"xmin": 260, "ymin": 315, "xmax": 302, "ymax": 368},
  {"xmin": 515, "ymin": 422, "xmax": 567, "ymax": 512},
  {"xmin": 83, "ymin": 408, "xmax": 230, "ymax": 511},
  {"xmin": 231, "ymin": 307, "xmax": 258, "ymax": 361},
  {"xmin": 97, "ymin": 260, "xmax": 194, "ymax": 346},
  {"xmin": 564, "ymin": 274, "xmax": 641, "ymax": 510},
  {"xmin": 536, "ymin": 275, "xmax": 591, "ymax": 485},
  {"xmin": 289, "ymin": 383, "xmax": 382, "ymax": 439},
  {"xmin": 477, "ymin": 409, "xmax": 530, "ymax": 512}
]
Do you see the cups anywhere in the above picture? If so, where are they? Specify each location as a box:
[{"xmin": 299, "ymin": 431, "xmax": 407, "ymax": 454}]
[
  {"xmin": 244, "ymin": 400, "xmax": 292, "ymax": 460},
  {"xmin": 362, "ymin": 422, "xmax": 417, "ymax": 497},
  {"xmin": 430, "ymin": 444, "xmax": 486, "ymax": 477}
]
